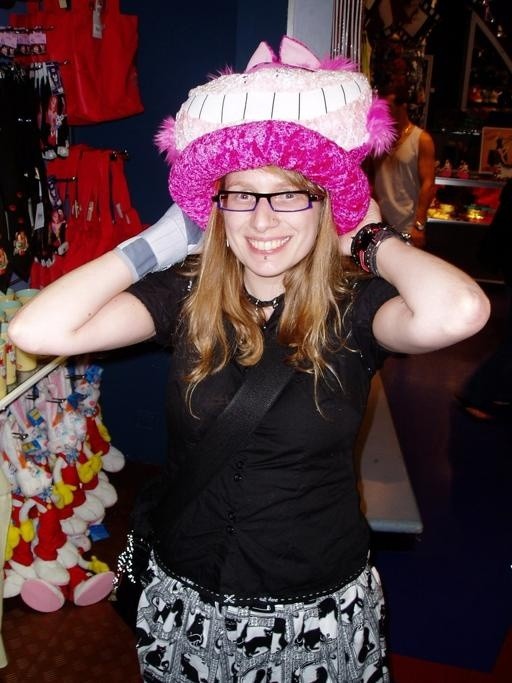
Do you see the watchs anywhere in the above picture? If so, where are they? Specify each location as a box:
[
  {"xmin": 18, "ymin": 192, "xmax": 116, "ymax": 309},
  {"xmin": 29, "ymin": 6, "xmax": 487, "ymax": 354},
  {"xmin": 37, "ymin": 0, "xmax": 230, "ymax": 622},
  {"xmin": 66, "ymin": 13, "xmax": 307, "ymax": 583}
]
[{"xmin": 415, "ymin": 220, "xmax": 427, "ymax": 232}]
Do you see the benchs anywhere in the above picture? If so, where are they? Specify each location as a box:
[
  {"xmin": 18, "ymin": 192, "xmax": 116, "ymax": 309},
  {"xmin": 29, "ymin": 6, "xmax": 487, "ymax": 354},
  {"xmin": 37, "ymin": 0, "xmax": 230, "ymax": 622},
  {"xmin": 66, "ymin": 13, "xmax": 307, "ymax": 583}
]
[{"xmin": 357, "ymin": 370, "xmax": 426, "ymax": 537}]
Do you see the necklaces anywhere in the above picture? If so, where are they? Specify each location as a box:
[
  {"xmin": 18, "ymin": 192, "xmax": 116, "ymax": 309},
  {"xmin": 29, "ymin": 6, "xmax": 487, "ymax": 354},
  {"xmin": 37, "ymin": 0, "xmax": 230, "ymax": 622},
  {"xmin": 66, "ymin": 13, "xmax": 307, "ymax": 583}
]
[
  {"xmin": 244, "ymin": 283, "xmax": 286, "ymax": 310},
  {"xmin": 396, "ymin": 121, "xmax": 411, "ymax": 145}
]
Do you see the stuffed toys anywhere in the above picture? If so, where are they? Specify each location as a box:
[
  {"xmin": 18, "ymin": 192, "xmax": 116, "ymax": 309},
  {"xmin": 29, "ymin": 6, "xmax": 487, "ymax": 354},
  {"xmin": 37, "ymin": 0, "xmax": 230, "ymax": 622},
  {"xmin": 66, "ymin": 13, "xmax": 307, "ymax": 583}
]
[{"xmin": 0, "ymin": 357, "xmax": 126, "ymax": 612}]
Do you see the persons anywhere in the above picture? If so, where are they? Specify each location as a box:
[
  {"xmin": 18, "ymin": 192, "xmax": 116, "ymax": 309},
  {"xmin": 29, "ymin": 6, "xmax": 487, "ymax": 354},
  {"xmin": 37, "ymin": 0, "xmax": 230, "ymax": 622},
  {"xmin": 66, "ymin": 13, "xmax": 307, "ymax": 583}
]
[
  {"xmin": 7, "ymin": 165, "xmax": 493, "ymax": 682},
  {"xmin": 373, "ymin": 80, "xmax": 439, "ymax": 252},
  {"xmin": 494, "ymin": 136, "xmax": 512, "ymax": 169}
]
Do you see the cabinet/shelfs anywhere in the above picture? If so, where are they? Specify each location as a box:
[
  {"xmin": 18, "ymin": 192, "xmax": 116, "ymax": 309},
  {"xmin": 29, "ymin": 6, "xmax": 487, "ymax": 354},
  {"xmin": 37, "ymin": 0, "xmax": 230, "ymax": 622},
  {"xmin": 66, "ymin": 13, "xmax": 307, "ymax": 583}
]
[
  {"xmin": 1, "ymin": 0, "xmax": 99, "ymax": 460},
  {"xmin": 427, "ymin": 165, "xmax": 507, "ymax": 232}
]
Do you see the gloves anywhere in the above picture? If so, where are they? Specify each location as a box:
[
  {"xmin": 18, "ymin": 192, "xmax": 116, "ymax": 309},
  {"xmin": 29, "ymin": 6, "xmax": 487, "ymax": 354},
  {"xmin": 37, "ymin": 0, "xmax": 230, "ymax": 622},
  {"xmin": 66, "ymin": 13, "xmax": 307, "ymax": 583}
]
[{"xmin": 114, "ymin": 192, "xmax": 209, "ymax": 280}]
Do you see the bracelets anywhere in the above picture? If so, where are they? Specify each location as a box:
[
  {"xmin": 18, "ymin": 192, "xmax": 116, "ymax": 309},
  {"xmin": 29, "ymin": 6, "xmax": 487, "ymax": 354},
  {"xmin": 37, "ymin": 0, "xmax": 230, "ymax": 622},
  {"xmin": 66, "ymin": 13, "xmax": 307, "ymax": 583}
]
[{"xmin": 350, "ymin": 220, "xmax": 413, "ymax": 278}]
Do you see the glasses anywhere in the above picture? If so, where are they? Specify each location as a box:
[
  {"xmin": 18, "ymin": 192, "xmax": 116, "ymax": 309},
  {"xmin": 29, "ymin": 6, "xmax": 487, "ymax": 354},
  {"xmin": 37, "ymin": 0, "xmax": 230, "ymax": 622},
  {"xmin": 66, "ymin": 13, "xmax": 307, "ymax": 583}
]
[{"xmin": 212, "ymin": 182, "xmax": 324, "ymax": 213}]
